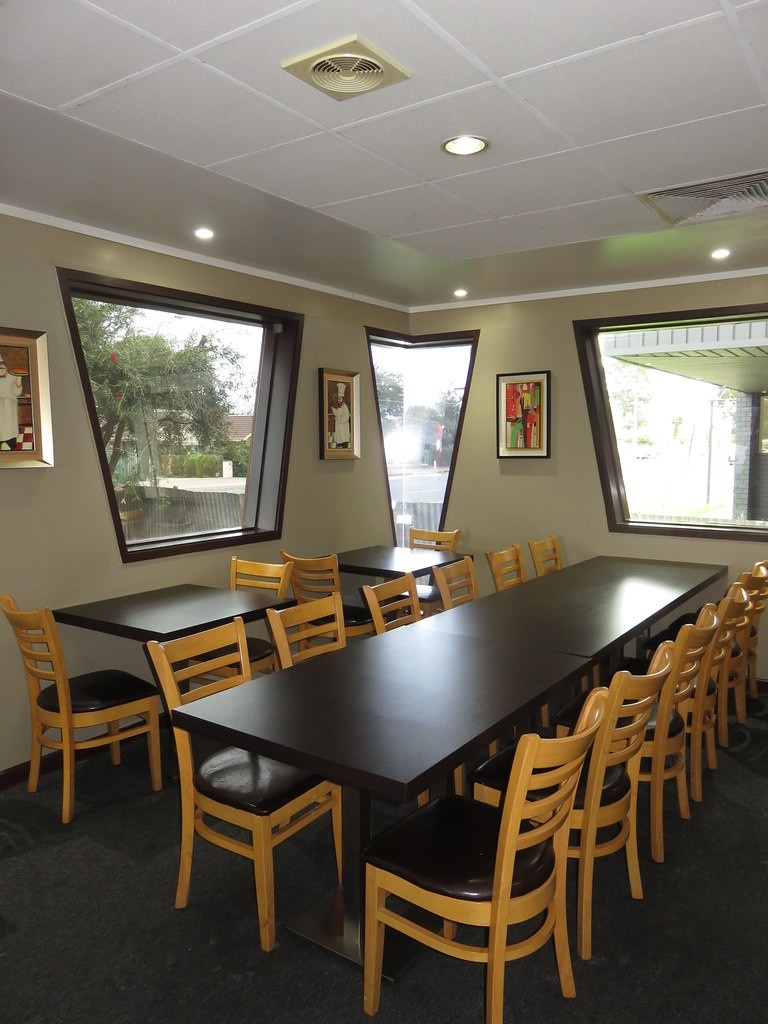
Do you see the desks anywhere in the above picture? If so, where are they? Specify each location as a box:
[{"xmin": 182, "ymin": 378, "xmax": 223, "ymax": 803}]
[
  {"xmin": 52, "ymin": 585, "xmax": 297, "ymax": 776},
  {"xmin": 167, "ymin": 556, "xmax": 728, "ymax": 984},
  {"xmin": 313, "ymin": 544, "xmax": 473, "ymax": 623}
]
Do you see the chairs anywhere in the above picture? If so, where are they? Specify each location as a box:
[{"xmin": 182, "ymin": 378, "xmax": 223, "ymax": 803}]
[
  {"xmin": 362, "ymin": 685, "xmax": 613, "ymax": 1023},
  {"xmin": 0, "ymin": 594, "xmax": 162, "ymax": 822},
  {"xmin": 395, "ymin": 528, "xmax": 462, "ymax": 617},
  {"xmin": 431, "ymin": 554, "xmax": 550, "ymax": 729},
  {"xmin": 280, "ymin": 548, "xmax": 388, "ymax": 652},
  {"xmin": 467, "ymin": 640, "xmax": 677, "ymax": 960},
  {"xmin": 145, "ymin": 615, "xmax": 344, "ymax": 953},
  {"xmin": 528, "ymin": 535, "xmax": 561, "ymax": 577},
  {"xmin": 487, "ymin": 544, "xmax": 600, "ymax": 703},
  {"xmin": 361, "ymin": 572, "xmax": 499, "ymax": 797},
  {"xmin": 612, "ymin": 562, "xmax": 768, "ymax": 863},
  {"xmin": 187, "ymin": 555, "xmax": 293, "ymax": 684},
  {"xmin": 266, "ymin": 591, "xmax": 430, "ymax": 807}
]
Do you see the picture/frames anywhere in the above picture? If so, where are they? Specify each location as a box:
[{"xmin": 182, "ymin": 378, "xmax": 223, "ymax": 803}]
[
  {"xmin": 318, "ymin": 367, "xmax": 362, "ymax": 461},
  {"xmin": 0, "ymin": 327, "xmax": 56, "ymax": 470},
  {"xmin": 495, "ymin": 370, "xmax": 550, "ymax": 459}
]
